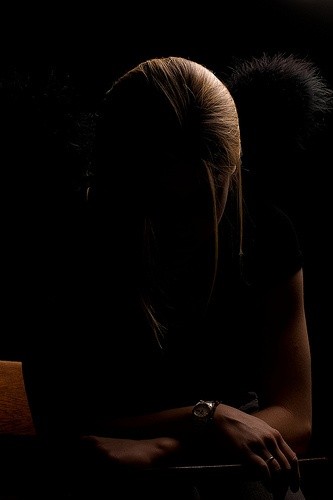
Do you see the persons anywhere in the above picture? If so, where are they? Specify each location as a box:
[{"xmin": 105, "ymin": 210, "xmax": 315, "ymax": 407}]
[
  {"xmin": 222, "ymin": 55, "xmax": 332, "ymax": 189},
  {"xmin": 20, "ymin": 56, "xmax": 313, "ymax": 499}
]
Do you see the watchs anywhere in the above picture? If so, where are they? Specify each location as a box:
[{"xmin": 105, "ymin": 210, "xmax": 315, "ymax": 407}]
[{"xmin": 192, "ymin": 400, "xmax": 220, "ymax": 431}]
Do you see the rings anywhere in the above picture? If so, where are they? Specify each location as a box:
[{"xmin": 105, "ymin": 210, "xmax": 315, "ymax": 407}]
[{"xmin": 265, "ymin": 456, "xmax": 274, "ymax": 465}]
